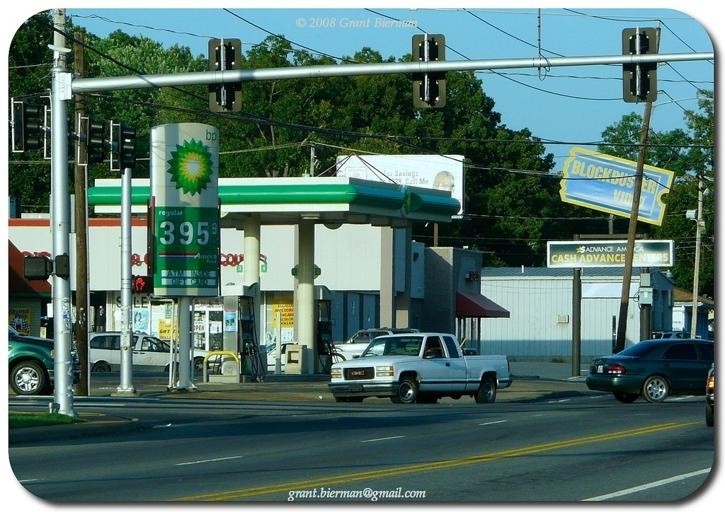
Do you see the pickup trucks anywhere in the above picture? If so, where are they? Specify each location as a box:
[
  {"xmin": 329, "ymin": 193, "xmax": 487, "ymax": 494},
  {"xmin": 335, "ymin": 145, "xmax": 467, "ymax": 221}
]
[{"xmin": 330, "ymin": 332, "xmax": 515, "ymax": 406}]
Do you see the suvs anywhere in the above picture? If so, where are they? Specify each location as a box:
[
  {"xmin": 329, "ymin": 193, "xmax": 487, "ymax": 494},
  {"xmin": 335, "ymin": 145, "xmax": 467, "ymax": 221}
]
[{"xmin": 8, "ymin": 323, "xmax": 82, "ymax": 395}]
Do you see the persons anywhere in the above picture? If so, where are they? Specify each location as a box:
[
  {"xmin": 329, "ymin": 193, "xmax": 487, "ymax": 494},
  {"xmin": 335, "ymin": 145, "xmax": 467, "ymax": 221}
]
[{"xmin": 432, "ymin": 172, "xmax": 455, "ymax": 196}]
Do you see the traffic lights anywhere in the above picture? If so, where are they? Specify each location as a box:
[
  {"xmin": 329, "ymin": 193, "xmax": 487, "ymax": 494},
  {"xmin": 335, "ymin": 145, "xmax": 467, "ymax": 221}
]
[
  {"xmin": 12, "ymin": 100, "xmax": 41, "ymax": 152},
  {"xmin": 87, "ymin": 119, "xmax": 104, "ymax": 163},
  {"xmin": 66, "ymin": 114, "xmax": 72, "ymax": 156},
  {"xmin": 118, "ymin": 124, "xmax": 136, "ymax": 169}
]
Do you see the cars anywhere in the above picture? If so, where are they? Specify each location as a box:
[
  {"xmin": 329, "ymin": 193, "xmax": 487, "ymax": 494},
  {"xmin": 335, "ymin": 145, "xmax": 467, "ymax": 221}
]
[
  {"xmin": 585, "ymin": 339, "xmax": 715, "ymax": 404},
  {"xmin": 651, "ymin": 330, "xmax": 694, "ymax": 340},
  {"xmin": 705, "ymin": 361, "xmax": 716, "ymax": 426},
  {"xmin": 266, "ymin": 342, "xmax": 298, "ymax": 371},
  {"xmin": 330, "ymin": 327, "xmax": 420, "ymax": 363},
  {"xmin": 131, "ymin": 337, "xmax": 221, "ymax": 367}
]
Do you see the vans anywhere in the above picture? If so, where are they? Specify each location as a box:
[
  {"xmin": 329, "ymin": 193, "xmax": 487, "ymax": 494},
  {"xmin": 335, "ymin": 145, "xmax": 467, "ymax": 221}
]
[{"xmin": 88, "ymin": 331, "xmax": 209, "ymax": 377}]
